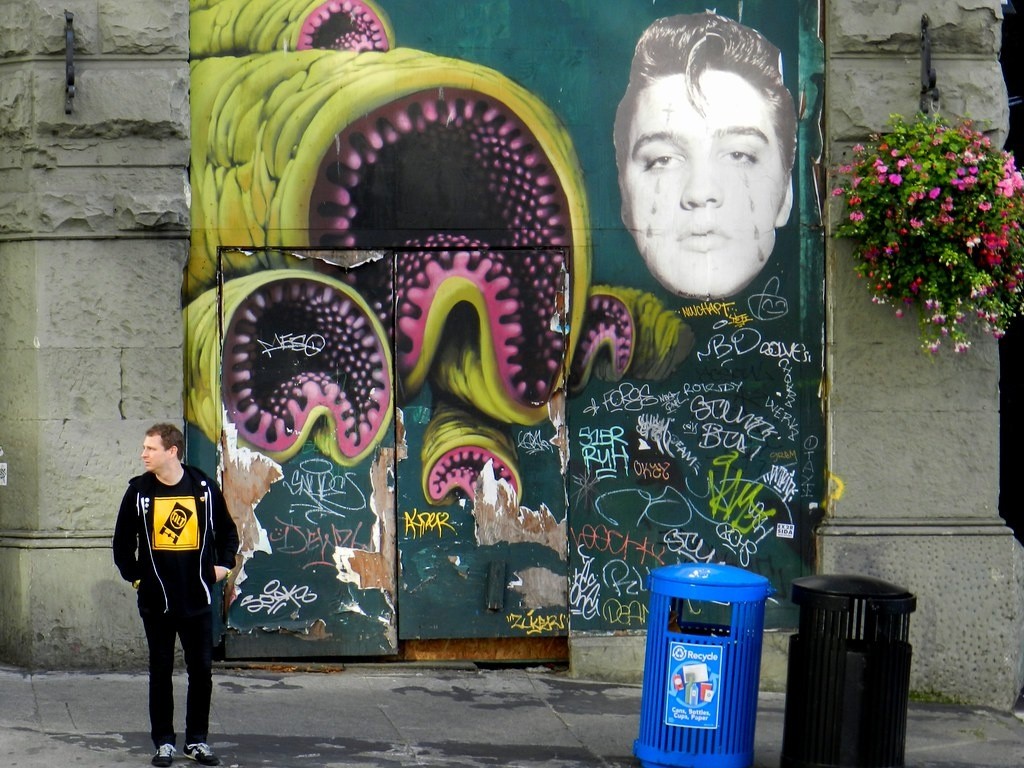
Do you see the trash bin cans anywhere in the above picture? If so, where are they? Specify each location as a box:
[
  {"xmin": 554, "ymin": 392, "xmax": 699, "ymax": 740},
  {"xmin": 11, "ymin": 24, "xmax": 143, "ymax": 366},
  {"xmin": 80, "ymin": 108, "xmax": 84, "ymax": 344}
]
[
  {"xmin": 632, "ymin": 562, "xmax": 778, "ymax": 768},
  {"xmin": 780, "ymin": 573, "xmax": 917, "ymax": 768}
]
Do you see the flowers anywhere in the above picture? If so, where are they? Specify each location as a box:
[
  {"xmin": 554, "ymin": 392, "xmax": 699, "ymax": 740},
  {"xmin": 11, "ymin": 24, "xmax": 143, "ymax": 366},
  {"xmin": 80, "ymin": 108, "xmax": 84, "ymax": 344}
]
[{"xmin": 820, "ymin": 100, "xmax": 1024, "ymax": 362}]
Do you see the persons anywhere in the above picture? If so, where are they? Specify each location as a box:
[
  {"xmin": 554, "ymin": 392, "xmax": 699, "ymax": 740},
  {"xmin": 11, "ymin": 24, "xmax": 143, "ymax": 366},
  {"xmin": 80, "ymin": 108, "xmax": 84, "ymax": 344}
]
[
  {"xmin": 613, "ymin": 12, "xmax": 797, "ymax": 301},
  {"xmin": 113, "ymin": 423, "xmax": 239, "ymax": 767}
]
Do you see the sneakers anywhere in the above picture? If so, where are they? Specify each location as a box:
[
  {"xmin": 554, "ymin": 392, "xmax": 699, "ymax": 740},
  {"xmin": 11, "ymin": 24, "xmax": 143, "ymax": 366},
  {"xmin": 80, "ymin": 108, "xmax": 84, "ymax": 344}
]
[
  {"xmin": 151, "ymin": 743, "xmax": 177, "ymax": 767},
  {"xmin": 183, "ymin": 743, "xmax": 220, "ymax": 766}
]
